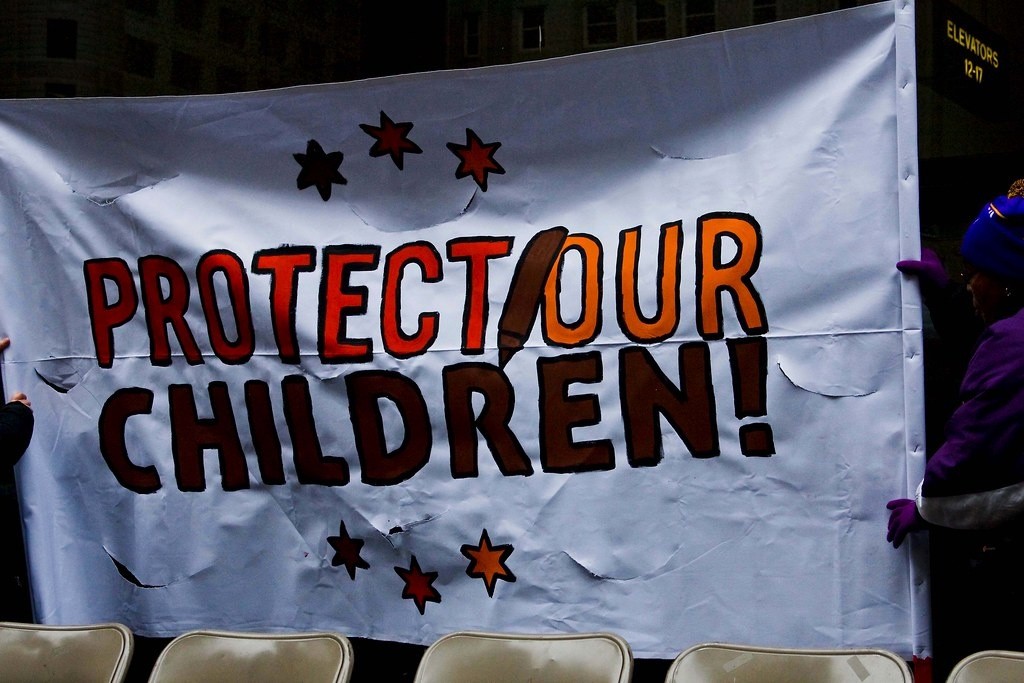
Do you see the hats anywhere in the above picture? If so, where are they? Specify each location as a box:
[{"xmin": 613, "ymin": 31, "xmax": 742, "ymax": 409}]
[{"xmin": 962, "ymin": 196, "xmax": 1024, "ymax": 280}]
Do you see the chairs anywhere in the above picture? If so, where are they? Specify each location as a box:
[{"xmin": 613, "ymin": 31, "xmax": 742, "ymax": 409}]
[
  {"xmin": 664, "ymin": 641, "xmax": 915, "ymax": 683},
  {"xmin": 412, "ymin": 629, "xmax": 634, "ymax": 683},
  {"xmin": 146, "ymin": 628, "xmax": 353, "ymax": 682},
  {"xmin": 0, "ymin": 621, "xmax": 132, "ymax": 683},
  {"xmin": 945, "ymin": 649, "xmax": 1023, "ymax": 683}
]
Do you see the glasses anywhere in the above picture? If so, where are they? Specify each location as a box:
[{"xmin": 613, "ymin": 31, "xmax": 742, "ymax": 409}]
[{"xmin": 959, "ymin": 270, "xmax": 976, "ymax": 283}]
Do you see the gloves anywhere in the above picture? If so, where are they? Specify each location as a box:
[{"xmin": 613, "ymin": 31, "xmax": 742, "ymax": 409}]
[
  {"xmin": 886, "ymin": 498, "xmax": 927, "ymax": 549},
  {"xmin": 896, "ymin": 249, "xmax": 947, "ymax": 283}
]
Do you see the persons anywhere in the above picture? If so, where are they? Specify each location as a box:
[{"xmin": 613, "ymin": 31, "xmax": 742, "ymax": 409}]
[
  {"xmin": 888, "ymin": 201, "xmax": 1024, "ymax": 652},
  {"xmin": 1, "ymin": 333, "xmax": 35, "ymax": 626}
]
